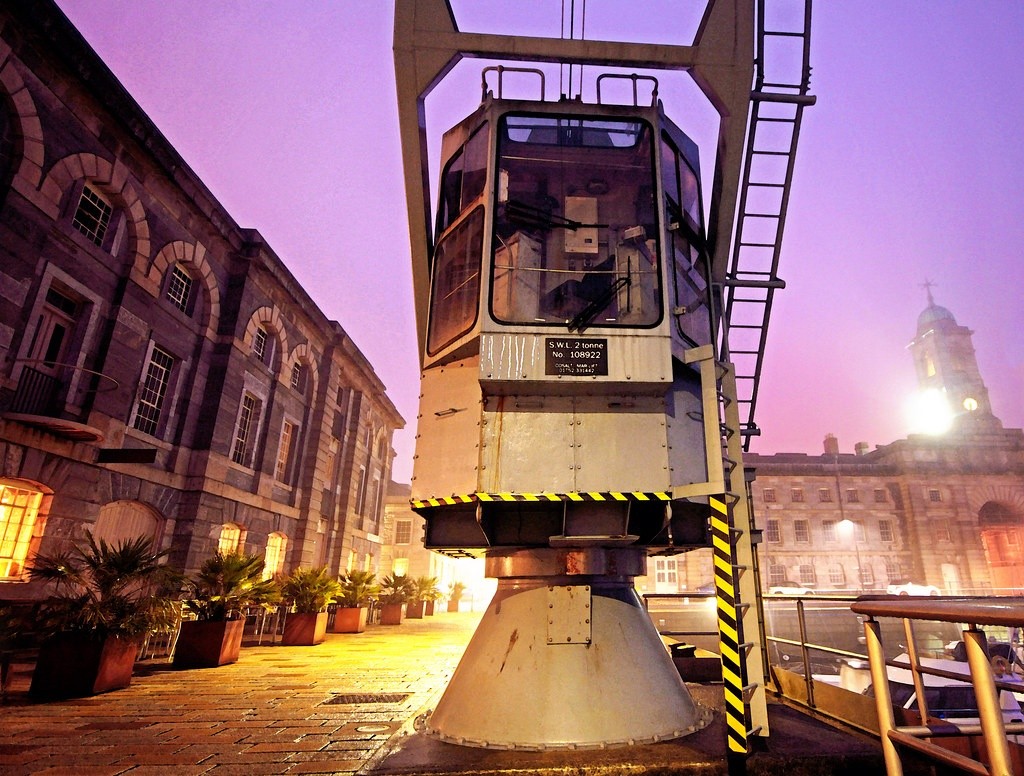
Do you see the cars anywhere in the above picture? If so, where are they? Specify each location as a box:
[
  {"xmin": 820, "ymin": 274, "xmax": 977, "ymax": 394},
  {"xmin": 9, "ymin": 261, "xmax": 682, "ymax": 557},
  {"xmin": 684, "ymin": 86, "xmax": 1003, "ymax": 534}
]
[
  {"xmin": 768, "ymin": 581, "xmax": 817, "ymax": 601},
  {"xmin": 887, "ymin": 579, "xmax": 941, "ymax": 597},
  {"xmin": 697, "ymin": 583, "xmax": 717, "ymax": 597}
]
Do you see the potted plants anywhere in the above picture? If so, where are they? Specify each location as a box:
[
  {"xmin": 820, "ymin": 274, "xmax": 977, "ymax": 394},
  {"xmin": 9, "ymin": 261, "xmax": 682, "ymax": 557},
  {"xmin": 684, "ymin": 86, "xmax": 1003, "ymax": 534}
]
[
  {"xmin": 19, "ymin": 528, "xmax": 191, "ymax": 696},
  {"xmin": 275, "ymin": 563, "xmax": 345, "ymax": 646},
  {"xmin": 332, "ymin": 568, "xmax": 385, "ymax": 634},
  {"xmin": 405, "ymin": 575, "xmax": 444, "ymax": 619},
  {"xmin": 172, "ymin": 545, "xmax": 287, "ymax": 668},
  {"xmin": 447, "ymin": 581, "xmax": 467, "ymax": 612},
  {"xmin": 373, "ymin": 570, "xmax": 419, "ymax": 625}
]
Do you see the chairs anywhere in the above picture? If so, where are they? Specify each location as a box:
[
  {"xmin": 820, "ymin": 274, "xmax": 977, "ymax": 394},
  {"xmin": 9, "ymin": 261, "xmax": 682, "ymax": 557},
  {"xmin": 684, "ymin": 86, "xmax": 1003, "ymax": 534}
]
[{"xmin": 167, "ymin": 599, "xmax": 199, "ymax": 662}]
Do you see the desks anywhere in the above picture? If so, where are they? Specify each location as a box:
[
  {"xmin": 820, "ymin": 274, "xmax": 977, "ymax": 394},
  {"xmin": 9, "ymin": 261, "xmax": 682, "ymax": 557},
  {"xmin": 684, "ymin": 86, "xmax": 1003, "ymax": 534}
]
[{"xmin": 246, "ymin": 605, "xmax": 282, "ymax": 645}]
